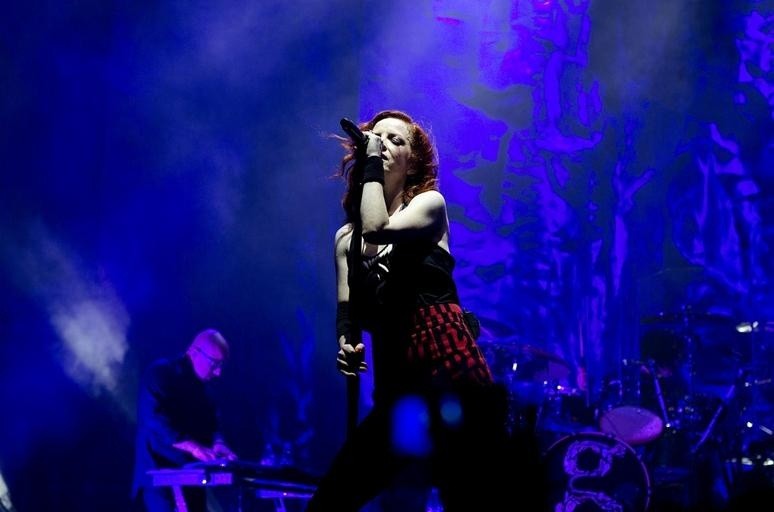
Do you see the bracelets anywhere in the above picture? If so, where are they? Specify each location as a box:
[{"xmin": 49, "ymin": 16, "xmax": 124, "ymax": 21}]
[{"xmin": 360, "ymin": 156, "xmax": 385, "ymax": 186}]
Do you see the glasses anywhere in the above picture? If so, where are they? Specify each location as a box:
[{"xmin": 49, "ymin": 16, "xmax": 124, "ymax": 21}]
[{"xmin": 197, "ymin": 348, "xmax": 227, "ymax": 370}]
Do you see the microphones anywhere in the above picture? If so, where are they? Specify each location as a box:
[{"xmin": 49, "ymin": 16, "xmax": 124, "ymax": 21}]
[{"xmin": 340, "ymin": 118, "xmax": 369, "ymax": 154}]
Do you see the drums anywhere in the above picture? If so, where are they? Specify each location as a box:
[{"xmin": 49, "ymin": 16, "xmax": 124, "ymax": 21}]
[
  {"xmin": 542, "ymin": 432, "xmax": 651, "ymax": 511},
  {"xmin": 599, "ymin": 361, "xmax": 663, "ymax": 444},
  {"xmin": 538, "ymin": 380, "xmax": 601, "ymax": 434}
]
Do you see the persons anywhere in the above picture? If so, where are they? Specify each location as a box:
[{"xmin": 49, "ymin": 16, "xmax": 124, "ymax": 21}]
[
  {"xmin": 131, "ymin": 328, "xmax": 237, "ymax": 510},
  {"xmin": 301, "ymin": 111, "xmax": 513, "ymax": 512}
]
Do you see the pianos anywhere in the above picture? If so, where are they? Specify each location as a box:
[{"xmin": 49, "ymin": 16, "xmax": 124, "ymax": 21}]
[{"xmin": 146, "ymin": 457, "xmax": 318, "ymax": 499}]
